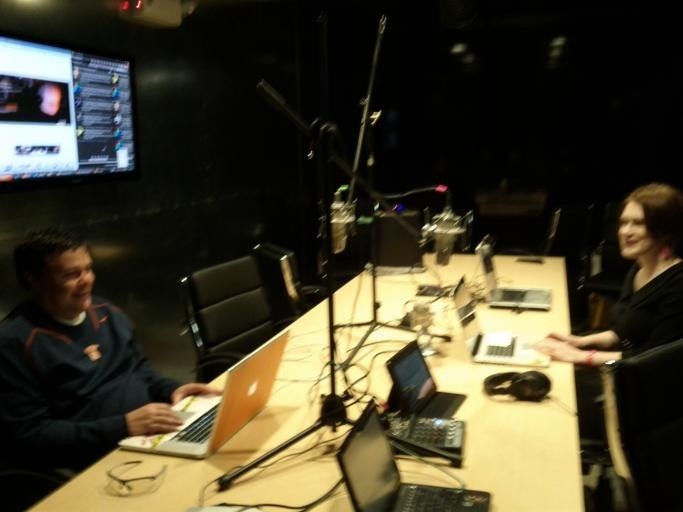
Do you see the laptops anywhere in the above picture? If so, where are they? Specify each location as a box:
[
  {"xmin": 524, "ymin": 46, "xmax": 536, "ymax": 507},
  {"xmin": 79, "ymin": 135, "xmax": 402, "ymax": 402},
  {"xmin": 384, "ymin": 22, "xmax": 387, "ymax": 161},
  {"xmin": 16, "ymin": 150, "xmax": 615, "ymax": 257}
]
[
  {"xmin": 478, "ymin": 245, "xmax": 552, "ymax": 311},
  {"xmin": 329, "ymin": 397, "xmax": 489, "ymax": 511},
  {"xmin": 450, "ymin": 277, "xmax": 553, "ymax": 368},
  {"xmin": 117, "ymin": 326, "xmax": 289, "ymax": 460}
]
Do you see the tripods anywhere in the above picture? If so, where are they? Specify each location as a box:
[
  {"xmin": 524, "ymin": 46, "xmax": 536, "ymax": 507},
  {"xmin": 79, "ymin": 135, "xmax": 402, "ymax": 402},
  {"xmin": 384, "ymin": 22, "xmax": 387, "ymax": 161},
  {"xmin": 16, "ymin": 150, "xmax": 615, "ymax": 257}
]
[
  {"xmin": 334, "ymin": 233, "xmax": 451, "ymax": 370},
  {"xmin": 214, "ymin": 170, "xmax": 462, "ymax": 492}
]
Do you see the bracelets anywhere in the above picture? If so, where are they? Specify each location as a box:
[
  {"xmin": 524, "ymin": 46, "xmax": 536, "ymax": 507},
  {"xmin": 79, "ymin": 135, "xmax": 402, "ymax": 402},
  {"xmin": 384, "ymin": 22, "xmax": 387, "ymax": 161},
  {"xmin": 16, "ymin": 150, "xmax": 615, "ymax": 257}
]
[{"xmin": 584, "ymin": 350, "xmax": 597, "ymax": 365}]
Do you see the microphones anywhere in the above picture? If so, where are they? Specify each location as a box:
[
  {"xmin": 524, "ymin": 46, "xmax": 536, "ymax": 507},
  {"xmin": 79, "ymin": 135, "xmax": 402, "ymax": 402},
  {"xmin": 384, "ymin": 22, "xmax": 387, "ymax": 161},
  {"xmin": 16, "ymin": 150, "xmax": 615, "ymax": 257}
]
[{"xmin": 426, "ymin": 213, "xmax": 466, "ymax": 266}]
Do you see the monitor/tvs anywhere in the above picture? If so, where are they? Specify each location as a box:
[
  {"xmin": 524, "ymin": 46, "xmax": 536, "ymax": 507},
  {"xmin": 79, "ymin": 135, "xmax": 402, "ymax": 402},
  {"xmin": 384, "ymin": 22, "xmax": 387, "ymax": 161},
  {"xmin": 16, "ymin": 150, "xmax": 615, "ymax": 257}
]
[{"xmin": 0, "ymin": 33, "xmax": 140, "ymax": 187}]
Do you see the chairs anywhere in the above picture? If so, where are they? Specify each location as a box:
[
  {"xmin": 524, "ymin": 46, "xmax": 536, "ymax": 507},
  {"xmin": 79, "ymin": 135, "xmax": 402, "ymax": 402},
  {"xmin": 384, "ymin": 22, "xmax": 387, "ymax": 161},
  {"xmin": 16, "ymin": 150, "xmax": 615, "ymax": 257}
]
[
  {"xmin": 581, "ymin": 368, "xmax": 641, "ymax": 511},
  {"xmin": 256, "ymin": 240, "xmax": 336, "ymax": 322},
  {"xmin": 179, "ymin": 257, "xmax": 304, "ymax": 383}
]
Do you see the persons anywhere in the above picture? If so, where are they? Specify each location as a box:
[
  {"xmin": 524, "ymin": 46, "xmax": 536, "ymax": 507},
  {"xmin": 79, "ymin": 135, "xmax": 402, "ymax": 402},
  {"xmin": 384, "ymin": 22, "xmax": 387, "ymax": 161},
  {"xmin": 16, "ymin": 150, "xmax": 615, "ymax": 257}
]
[
  {"xmin": 0, "ymin": 222, "xmax": 224, "ymax": 475},
  {"xmin": 36, "ymin": 84, "xmax": 61, "ymax": 117},
  {"xmin": 530, "ymin": 182, "xmax": 682, "ymax": 369}
]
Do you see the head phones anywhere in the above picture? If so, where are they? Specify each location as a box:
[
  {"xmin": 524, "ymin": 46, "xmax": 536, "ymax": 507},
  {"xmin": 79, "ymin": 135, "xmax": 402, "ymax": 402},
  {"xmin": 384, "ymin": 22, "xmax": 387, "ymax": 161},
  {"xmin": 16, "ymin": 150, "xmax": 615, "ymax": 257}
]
[{"xmin": 484, "ymin": 372, "xmax": 549, "ymax": 400}]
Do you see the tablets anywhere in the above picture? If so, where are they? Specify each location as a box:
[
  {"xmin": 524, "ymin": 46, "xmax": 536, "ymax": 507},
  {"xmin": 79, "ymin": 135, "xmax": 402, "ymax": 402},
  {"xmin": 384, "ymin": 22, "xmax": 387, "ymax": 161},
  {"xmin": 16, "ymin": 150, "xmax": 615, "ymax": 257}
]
[{"xmin": 385, "ymin": 339, "xmax": 436, "ymax": 414}]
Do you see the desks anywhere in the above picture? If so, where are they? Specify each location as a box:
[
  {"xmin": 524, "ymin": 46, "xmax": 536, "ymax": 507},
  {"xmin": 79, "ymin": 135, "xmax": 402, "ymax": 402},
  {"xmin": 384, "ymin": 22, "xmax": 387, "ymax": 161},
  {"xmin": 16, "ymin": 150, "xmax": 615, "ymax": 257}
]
[{"xmin": 22, "ymin": 253, "xmax": 588, "ymax": 512}]
